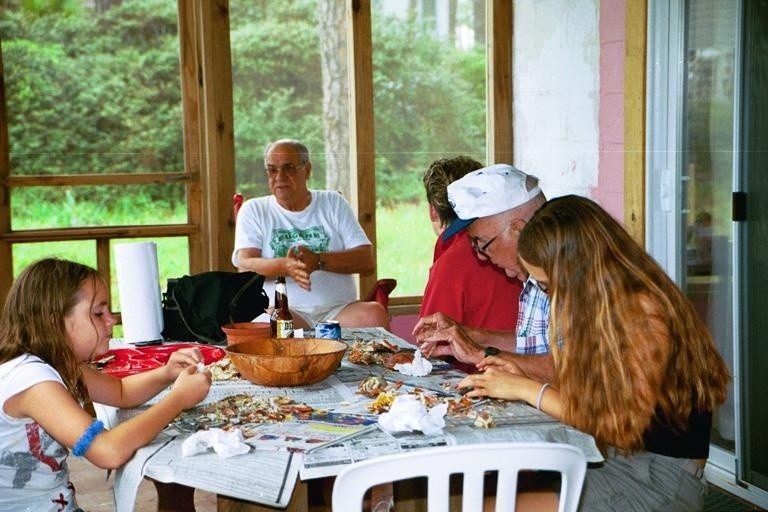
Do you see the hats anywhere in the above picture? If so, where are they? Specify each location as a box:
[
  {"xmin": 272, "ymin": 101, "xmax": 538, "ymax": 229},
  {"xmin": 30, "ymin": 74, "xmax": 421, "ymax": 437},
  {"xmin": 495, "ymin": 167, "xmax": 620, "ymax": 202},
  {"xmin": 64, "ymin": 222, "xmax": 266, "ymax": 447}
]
[{"xmin": 438, "ymin": 161, "xmax": 543, "ymax": 239}]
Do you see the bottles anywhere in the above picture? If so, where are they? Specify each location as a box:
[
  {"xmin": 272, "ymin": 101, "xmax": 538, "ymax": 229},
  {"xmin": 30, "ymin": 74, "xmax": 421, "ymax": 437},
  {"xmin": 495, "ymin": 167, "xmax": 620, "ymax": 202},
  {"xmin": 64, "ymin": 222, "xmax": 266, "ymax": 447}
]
[{"xmin": 270, "ymin": 277, "xmax": 294, "ymax": 338}]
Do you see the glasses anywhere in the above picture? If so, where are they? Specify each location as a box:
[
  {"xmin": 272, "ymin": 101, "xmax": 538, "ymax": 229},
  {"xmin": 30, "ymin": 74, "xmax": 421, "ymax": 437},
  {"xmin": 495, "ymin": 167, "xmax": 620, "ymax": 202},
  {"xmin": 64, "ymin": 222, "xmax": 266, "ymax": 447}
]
[{"xmin": 466, "ymin": 225, "xmax": 518, "ymax": 258}]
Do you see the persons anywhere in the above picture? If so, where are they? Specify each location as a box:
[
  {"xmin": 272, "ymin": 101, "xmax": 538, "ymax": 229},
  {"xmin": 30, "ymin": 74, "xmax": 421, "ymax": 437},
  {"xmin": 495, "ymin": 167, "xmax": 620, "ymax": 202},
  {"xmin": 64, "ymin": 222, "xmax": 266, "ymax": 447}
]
[
  {"xmin": 455, "ymin": 195, "xmax": 733, "ymax": 511},
  {"xmin": 1, "ymin": 258, "xmax": 211, "ymax": 511},
  {"xmin": 419, "ymin": 155, "xmax": 522, "ymax": 378},
  {"xmin": 411, "ymin": 163, "xmax": 565, "ymax": 382},
  {"xmin": 229, "ymin": 139, "xmax": 393, "ymax": 336}
]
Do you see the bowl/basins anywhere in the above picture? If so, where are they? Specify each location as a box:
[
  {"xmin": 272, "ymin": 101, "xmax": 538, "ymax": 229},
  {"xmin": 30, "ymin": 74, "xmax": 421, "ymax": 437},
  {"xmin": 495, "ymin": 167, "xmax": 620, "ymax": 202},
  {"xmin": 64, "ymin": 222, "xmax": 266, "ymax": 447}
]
[
  {"xmin": 223, "ymin": 338, "xmax": 349, "ymax": 385},
  {"xmin": 218, "ymin": 321, "xmax": 272, "ymax": 343}
]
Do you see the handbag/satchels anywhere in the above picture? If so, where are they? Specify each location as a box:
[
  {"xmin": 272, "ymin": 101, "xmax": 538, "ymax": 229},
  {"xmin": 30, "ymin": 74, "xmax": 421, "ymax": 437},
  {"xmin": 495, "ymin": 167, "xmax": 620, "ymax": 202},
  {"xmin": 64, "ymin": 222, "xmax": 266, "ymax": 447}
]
[{"xmin": 161, "ymin": 270, "xmax": 269, "ymax": 346}]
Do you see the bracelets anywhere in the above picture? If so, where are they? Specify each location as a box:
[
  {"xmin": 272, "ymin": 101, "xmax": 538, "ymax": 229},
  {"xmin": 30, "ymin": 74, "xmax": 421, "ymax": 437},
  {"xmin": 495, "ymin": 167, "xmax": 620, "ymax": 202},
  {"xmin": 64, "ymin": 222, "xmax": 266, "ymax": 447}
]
[{"xmin": 536, "ymin": 382, "xmax": 550, "ymax": 411}]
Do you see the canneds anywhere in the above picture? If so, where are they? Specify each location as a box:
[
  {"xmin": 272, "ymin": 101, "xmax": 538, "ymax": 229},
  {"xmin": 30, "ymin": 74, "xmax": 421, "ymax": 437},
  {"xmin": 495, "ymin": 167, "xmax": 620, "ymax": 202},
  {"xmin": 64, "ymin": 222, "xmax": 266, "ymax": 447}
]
[{"xmin": 316, "ymin": 320, "xmax": 341, "ymax": 367}]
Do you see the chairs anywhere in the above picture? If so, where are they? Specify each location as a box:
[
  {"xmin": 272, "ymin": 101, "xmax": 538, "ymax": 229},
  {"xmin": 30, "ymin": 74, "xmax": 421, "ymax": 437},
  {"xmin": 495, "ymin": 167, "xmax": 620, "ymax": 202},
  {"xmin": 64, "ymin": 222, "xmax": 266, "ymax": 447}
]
[{"xmin": 329, "ymin": 442, "xmax": 589, "ymax": 512}]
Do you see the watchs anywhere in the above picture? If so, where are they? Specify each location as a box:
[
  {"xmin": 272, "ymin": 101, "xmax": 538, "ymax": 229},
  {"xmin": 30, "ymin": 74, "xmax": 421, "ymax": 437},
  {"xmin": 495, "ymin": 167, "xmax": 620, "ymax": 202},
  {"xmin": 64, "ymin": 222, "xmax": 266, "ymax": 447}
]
[
  {"xmin": 484, "ymin": 346, "xmax": 501, "ymax": 358},
  {"xmin": 316, "ymin": 251, "xmax": 326, "ymax": 271}
]
